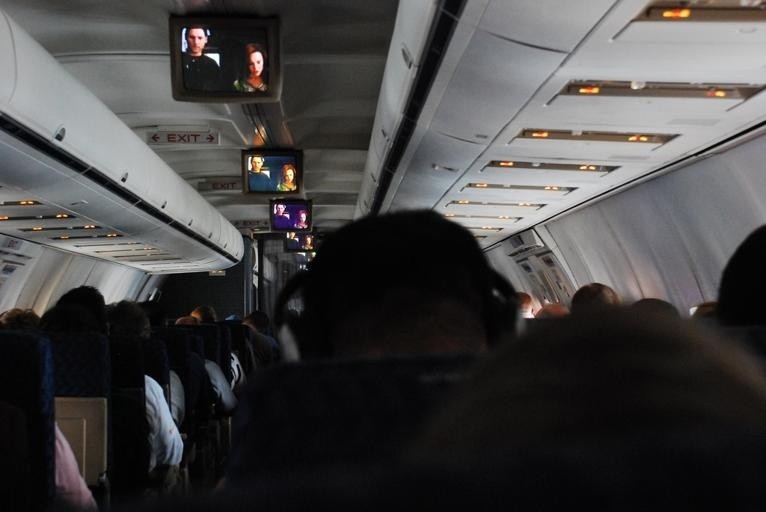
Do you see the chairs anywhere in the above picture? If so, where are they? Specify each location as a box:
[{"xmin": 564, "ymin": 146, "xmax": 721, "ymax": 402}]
[{"xmin": 55, "ymin": 321, "xmax": 250, "ymax": 508}]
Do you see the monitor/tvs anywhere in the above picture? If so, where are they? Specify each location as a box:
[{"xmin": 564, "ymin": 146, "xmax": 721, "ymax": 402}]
[
  {"xmin": 283, "ymin": 231, "xmax": 316, "ymax": 253},
  {"xmin": 166, "ymin": 9, "xmax": 282, "ymax": 105},
  {"xmin": 269, "ymin": 197, "xmax": 312, "ymax": 233},
  {"xmin": 242, "ymin": 148, "xmax": 304, "ymax": 204}
]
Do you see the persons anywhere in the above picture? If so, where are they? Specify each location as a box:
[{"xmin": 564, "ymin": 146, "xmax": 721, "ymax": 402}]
[
  {"xmin": 182, "ymin": 26, "xmax": 224, "ymax": 92},
  {"xmin": 248, "ymin": 155, "xmax": 275, "ymax": 192},
  {"xmin": 2, "ymin": 285, "xmax": 269, "ymax": 512},
  {"xmin": 275, "ymin": 203, "xmax": 291, "ymax": 229},
  {"xmin": 234, "ymin": 211, "xmax": 766, "ymax": 462},
  {"xmin": 233, "ymin": 43, "xmax": 269, "ymax": 93},
  {"xmin": 277, "ymin": 162, "xmax": 296, "ymax": 192}
]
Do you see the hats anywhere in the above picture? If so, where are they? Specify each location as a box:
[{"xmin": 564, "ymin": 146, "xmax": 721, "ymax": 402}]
[{"xmin": 300, "ymin": 209, "xmax": 520, "ymax": 333}]
